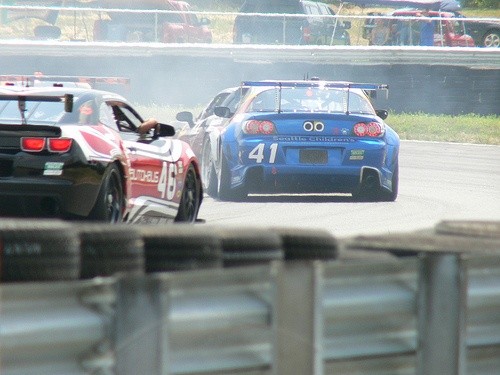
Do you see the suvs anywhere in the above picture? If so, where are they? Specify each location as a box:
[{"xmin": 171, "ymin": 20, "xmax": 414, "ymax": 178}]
[
  {"xmin": 362, "ymin": 8, "xmax": 499, "ymax": 47},
  {"xmin": 381, "ymin": 9, "xmax": 475, "ymax": 46}
]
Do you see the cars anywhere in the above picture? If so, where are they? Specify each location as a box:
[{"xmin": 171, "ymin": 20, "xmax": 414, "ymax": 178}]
[{"xmin": 93, "ymin": 0, "xmax": 212, "ymax": 42}]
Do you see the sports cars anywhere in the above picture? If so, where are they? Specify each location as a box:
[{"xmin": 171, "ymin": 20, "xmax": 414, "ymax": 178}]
[
  {"xmin": 176, "ymin": 77, "xmax": 400, "ymax": 202},
  {"xmin": 0, "ymin": 82, "xmax": 204, "ymax": 222}
]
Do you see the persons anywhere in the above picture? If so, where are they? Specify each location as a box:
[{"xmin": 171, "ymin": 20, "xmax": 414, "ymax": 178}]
[{"xmin": 134, "ymin": 120, "xmax": 160, "ymax": 134}]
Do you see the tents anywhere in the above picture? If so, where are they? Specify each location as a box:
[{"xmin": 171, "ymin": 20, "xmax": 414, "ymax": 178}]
[{"xmin": 330, "ymin": 0, "xmax": 469, "ymax": 47}]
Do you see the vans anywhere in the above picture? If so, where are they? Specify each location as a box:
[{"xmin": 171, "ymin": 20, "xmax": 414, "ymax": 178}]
[{"xmin": 233, "ymin": 0, "xmax": 352, "ymax": 45}]
